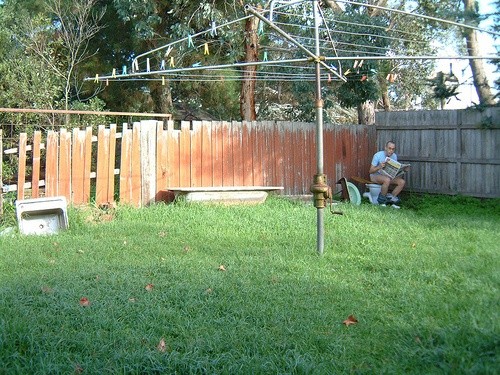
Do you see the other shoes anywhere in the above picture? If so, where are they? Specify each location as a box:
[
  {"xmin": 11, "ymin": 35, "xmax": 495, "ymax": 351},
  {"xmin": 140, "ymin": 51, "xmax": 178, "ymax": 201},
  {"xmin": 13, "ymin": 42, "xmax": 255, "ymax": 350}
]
[
  {"xmin": 391, "ymin": 204, "xmax": 400, "ymax": 209},
  {"xmin": 380, "ymin": 204, "xmax": 386, "ymax": 207}
]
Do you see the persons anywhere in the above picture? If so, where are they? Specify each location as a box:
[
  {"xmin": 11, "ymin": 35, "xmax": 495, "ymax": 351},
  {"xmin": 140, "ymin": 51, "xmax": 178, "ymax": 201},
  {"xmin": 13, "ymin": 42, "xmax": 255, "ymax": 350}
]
[{"xmin": 369, "ymin": 141, "xmax": 409, "ymax": 207}]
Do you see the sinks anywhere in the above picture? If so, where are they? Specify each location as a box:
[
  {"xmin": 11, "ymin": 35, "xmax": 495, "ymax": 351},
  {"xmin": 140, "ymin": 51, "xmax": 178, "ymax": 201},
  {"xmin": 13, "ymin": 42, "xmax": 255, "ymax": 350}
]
[
  {"xmin": 15, "ymin": 196, "xmax": 70, "ymax": 237},
  {"xmin": 335, "ymin": 176, "xmax": 361, "ymax": 207}
]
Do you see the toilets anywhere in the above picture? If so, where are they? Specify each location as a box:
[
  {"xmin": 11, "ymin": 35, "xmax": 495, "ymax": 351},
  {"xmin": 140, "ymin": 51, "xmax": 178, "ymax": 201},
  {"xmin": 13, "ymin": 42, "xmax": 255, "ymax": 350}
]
[{"xmin": 362, "ymin": 183, "xmax": 391, "ymax": 206}]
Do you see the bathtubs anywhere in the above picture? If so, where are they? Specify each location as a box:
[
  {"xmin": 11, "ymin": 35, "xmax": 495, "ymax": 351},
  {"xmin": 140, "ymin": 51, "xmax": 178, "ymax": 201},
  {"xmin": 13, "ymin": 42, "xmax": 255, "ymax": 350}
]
[{"xmin": 163, "ymin": 185, "xmax": 285, "ymax": 205}]
